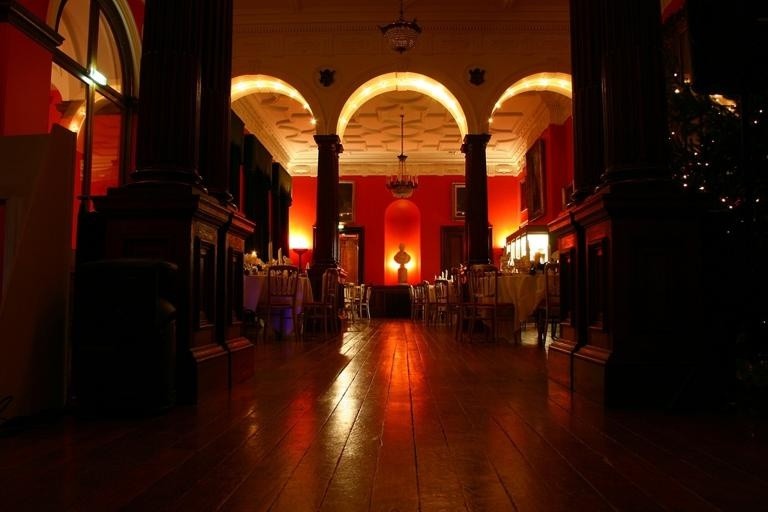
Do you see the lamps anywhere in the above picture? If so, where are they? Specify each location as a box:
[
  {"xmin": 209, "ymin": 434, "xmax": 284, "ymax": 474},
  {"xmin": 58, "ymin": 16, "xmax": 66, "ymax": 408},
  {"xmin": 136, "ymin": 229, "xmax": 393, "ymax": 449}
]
[
  {"xmin": 375, "ymin": 0, "xmax": 422, "ymax": 53},
  {"xmin": 293, "ymin": 247, "xmax": 307, "ymax": 271},
  {"xmin": 386, "ymin": 115, "xmax": 421, "ymax": 199}
]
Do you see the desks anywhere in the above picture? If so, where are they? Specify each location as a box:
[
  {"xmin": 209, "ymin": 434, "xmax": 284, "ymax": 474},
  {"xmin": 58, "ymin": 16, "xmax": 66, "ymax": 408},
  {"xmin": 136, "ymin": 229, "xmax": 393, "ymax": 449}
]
[{"xmin": 369, "ymin": 283, "xmax": 419, "ymax": 319}]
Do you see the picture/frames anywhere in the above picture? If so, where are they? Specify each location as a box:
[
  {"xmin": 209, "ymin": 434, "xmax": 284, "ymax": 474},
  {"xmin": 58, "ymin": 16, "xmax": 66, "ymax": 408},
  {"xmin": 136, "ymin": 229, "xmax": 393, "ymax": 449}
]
[
  {"xmin": 524, "ymin": 137, "xmax": 545, "ymax": 224},
  {"xmin": 336, "ymin": 179, "xmax": 355, "ymax": 223},
  {"xmin": 452, "ymin": 182, "xmax": 471, "ymax": 220}
]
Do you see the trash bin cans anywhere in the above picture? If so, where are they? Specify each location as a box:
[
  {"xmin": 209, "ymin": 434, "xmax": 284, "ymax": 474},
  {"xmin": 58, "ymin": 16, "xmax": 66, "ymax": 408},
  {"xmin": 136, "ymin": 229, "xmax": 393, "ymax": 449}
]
[{"xmin": 521, "ymin": 324, "xmax": 539, "ymax": 347}]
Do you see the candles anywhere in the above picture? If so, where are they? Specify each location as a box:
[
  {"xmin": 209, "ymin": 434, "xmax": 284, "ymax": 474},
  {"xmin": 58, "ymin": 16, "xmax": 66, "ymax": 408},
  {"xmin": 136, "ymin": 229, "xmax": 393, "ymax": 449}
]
[
  {"xmin": 268, "ymin": 242, "xmax": 273, "ymax": 263},
  {"xmin": 278, "ymin": 248, "xmax": 282, "ymax": 262}
]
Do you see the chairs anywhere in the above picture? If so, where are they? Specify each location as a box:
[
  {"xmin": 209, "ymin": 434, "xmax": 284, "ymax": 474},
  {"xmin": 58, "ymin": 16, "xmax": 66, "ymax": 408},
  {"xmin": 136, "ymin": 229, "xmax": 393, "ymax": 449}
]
[
  {"xmin": 240, "ymin": 262, "xmax": 377, "ymax": 342},
  {"xmin": 405, "ymin": 258, "xmax": 568, "ymax": 349}
]
